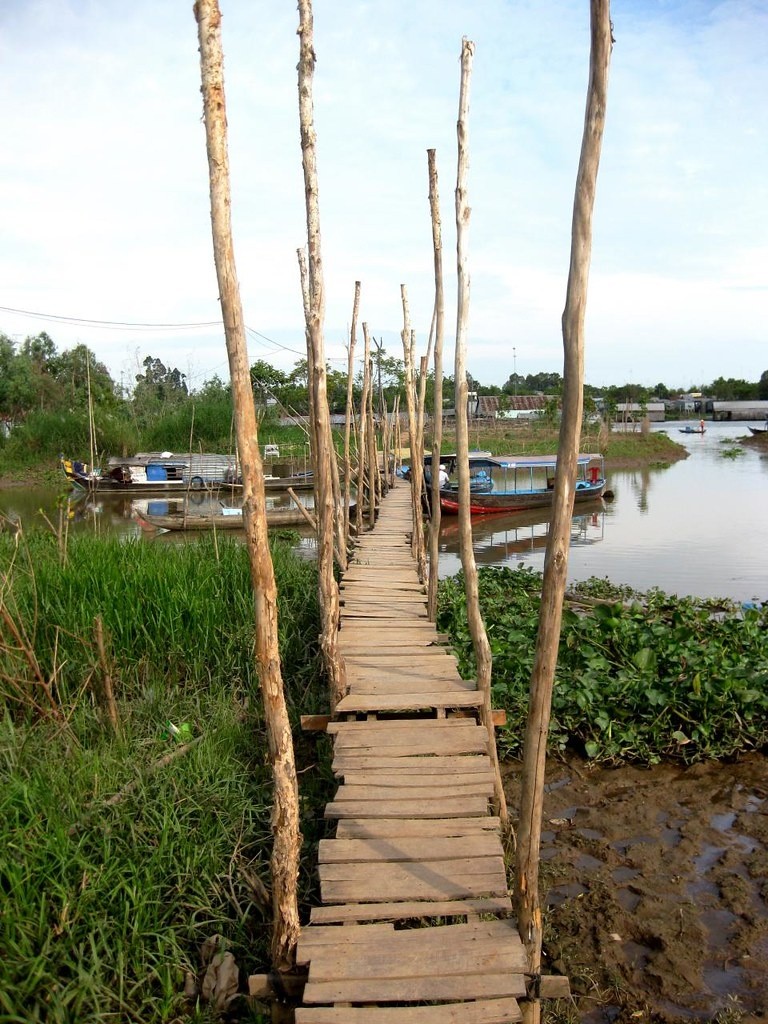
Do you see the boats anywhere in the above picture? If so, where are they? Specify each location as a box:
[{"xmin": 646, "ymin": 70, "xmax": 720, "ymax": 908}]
[
  {"xmin": 424, "ymin": 448, "xmax": 607, "ymax": 515},
  {"xmin": 218, "ymin": 467, "xmax": 360, "ymax": 493},
  {"xmin": 678, "ymin": 426, "xmax": 707, "ymax": 434},
  {"xmin": 134, "ymin": 503, "xmax": 358, "ymax": 531},
  {"xmin": 59, "ymin": 450, "xmax": 241, "ymax": 493},
  {"xmin": 745, "ymin": 425, "xmax": 768, "ymax": 436}
]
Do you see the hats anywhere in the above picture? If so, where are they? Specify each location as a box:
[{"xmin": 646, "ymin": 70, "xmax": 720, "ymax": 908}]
[{"xmin": 439, "ymin": 464, "xmax": 446, "ymax": 470}]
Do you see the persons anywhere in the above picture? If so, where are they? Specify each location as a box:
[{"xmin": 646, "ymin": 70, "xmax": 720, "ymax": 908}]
[
  {"xmin": 439, "ymin": 464, "xmax": 452, "ymax": 490},
  {"xmin": 699, "ymin": 420, "xmax": 705, "ymax": 431}
]
